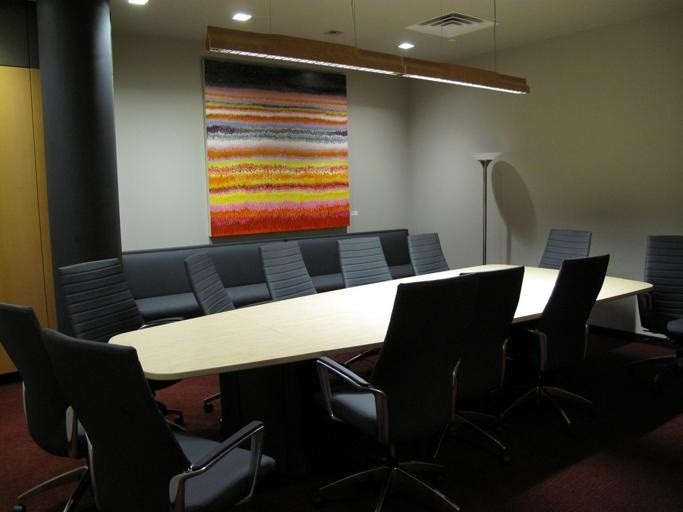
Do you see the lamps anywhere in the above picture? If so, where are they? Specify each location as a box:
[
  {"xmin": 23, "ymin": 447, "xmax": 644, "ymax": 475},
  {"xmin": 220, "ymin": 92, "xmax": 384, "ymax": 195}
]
[
  {"xmin": 204, "ymin": 0, "xmax": 530, "ymax": 94},
  {"xmin": 470, "ymin": 150, "xmax": 500, "ymax": 266}
]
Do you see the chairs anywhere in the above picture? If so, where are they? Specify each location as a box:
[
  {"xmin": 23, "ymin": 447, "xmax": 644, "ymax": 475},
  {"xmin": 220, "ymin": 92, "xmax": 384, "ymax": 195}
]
[
  {"xmin": 499, "ymin": 251, "xmax": 612, "ymax": 423},
  {"xmin": 426, "ymin": 263, "xmax": 529, "ymax": 466},
  {"xmin": 626, "ymin": 233, "xmax": 680, "ymax": 395},
  {"xmin": 52, "ymin": 256, "xmax": 192, "ymax": 392},
  {"xmin": 308, "ymin": 272, "xmax": 499, "ymax": 511},
  {"xmin": 179, "ymin": 250, "xmax": 245, "ymax": 415},
  {"xmin": 2, "ymin": 300, "xmax": 98, "ymax": 508},
  {"xmin": 36, "ymin": 326, "xmax": 279, "ymax": 508},
  {"xmin": 405, "ymin": 231, "xmax": 453, "ymax": 276},
  {"xmin": 336, "ymin": 235, "xmax": 394, "ymax": 370},
  {"xmin": 536, "ymin": 227, "xmax": 595, "ymax": 269},
  {"xmin": 256, "ymin": 239, "xmax": 322, "ymax": 302}
]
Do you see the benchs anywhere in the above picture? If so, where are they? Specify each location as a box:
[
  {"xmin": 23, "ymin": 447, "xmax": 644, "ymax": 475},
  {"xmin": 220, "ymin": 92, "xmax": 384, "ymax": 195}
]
[{"xmin": 120, "ymin": 228, "xmax": 419, "ymax": 325}]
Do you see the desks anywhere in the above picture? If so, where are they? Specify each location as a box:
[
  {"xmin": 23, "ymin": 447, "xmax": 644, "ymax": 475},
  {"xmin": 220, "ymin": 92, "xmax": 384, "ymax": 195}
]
[{"xmin": 103, "ymin": 259, "xmax": 657, "ymax": 429}]
